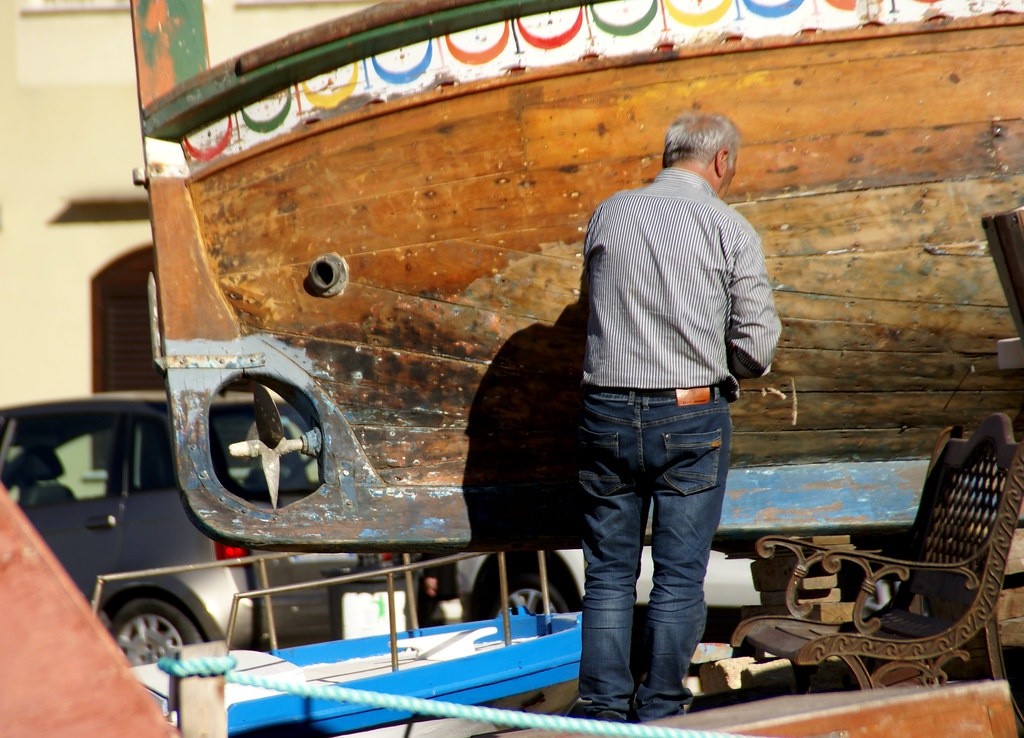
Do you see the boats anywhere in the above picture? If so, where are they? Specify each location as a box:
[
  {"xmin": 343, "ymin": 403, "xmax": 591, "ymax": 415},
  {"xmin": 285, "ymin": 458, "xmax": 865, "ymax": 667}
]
[
  {"xmin": 128, "ymin": 0, "xmax": 1024, "ymax": 551},
  {"xmin": 91, "ymin": 543, "xmax": 593, "ymax": 738}
]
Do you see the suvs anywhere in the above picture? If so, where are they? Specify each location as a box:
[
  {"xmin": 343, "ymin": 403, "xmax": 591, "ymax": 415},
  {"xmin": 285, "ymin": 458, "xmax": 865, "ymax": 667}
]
[{"xmin": 0, "ymin": 389, "xmax": 397, "ymax": 667}]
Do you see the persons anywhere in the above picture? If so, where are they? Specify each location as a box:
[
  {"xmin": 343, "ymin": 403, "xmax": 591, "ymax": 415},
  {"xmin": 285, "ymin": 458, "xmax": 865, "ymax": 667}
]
[{"xmin": 561, "ymin": 115, "xmax": 783, "ymax": 725}]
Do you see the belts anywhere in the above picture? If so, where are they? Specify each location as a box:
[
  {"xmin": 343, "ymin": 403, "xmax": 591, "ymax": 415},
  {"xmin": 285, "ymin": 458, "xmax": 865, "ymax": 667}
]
[{"xmin": 585, "ymin": 384, "xmax": 675, "ymax": 397}]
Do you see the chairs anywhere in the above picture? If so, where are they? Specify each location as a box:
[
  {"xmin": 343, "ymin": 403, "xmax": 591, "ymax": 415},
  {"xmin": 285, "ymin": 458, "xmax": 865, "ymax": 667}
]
[
  {"xmin": 731, "ymin": 411, "xmax": 1024, "ymax": 738},
  {"xmin": 11, "ymin": 446, "xmax": 74, "ymax": 508}
]
